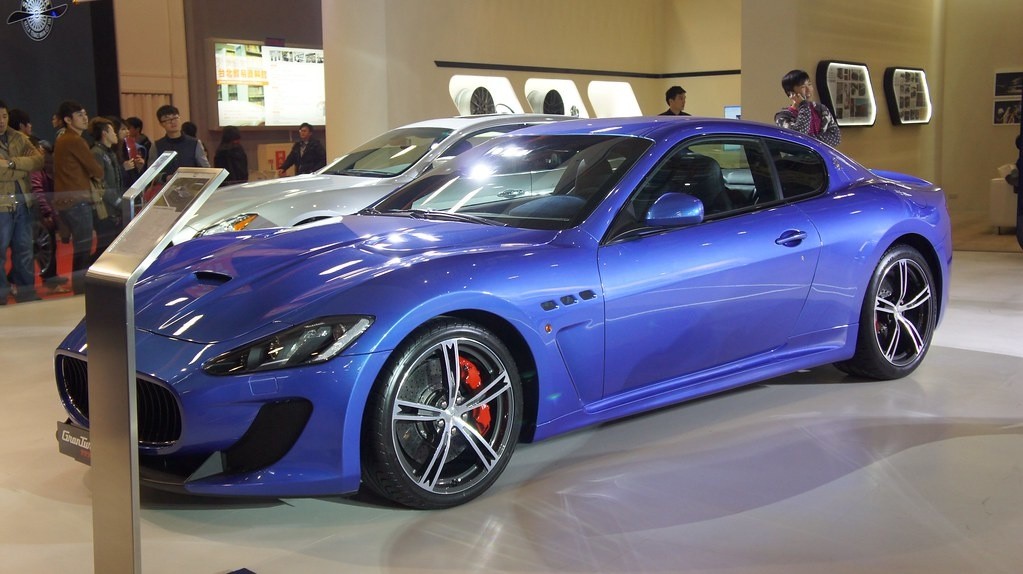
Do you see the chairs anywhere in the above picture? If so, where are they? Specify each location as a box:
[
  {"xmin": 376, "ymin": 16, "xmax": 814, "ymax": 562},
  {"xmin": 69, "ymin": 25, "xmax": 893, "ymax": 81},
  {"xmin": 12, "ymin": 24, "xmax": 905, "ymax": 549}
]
[
  {"xmin": 570, "ymin": 158, "xmax": 615, "ymax": 201},
  {"xmin": 423, "ymin": 137, "xmax": 473, "ymax": 170},
  {"xmin": 658, "ymin": 154, "xmax": 733, "ymax": 214}
]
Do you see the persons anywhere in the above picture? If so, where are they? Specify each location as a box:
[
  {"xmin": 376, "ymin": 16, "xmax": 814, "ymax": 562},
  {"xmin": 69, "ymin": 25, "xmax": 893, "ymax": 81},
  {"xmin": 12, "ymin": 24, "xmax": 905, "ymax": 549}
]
[
  {"xmin": 278, "ymin": 123, "xmax": 326, "ymax": 176},
  {"xmin": 657, "ymin": 86, "xmax": 693, "ymax": 149},
  {"xmin": 1005, "ymin": 134, "xmax": 1023, "ymax": 250},
  {"xmin": 0, "ymin": 102, "xmax": 210, "ymax": 306},
  {"xmin": 775, "ymin": 69, "xmax": 842, "ymax": 162},
  {"xmin": 214, "ymin": 126, "xmax": 248, "ymax": 186}
]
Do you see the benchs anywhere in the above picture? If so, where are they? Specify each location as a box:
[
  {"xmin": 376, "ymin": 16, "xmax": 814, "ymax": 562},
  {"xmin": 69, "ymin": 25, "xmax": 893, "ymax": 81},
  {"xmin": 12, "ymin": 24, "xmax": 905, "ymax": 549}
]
[{"xmin": 722, "ymin": 160, "xmax": 826, "ymax": 206}]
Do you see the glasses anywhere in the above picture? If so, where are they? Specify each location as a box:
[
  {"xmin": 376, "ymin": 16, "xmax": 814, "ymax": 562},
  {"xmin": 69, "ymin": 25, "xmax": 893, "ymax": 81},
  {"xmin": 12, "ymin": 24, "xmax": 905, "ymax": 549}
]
[{"xmin": 161, "ymin": 116, "xmax": 180, "ymax": 124}]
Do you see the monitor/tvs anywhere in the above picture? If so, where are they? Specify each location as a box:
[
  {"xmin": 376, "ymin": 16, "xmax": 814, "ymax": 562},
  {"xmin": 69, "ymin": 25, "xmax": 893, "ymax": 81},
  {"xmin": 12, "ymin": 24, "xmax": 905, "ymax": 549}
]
[{"xmin": 723, "ymin": 105, "xmax": 742, "ymax": 151}]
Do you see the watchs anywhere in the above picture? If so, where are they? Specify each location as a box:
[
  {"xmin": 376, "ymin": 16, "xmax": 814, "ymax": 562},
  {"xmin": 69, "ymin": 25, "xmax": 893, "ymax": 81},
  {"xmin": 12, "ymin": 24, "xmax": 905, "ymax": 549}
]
[{"xmin": 8, "ymin": 160, "xmax": 13, "ymax": 167}]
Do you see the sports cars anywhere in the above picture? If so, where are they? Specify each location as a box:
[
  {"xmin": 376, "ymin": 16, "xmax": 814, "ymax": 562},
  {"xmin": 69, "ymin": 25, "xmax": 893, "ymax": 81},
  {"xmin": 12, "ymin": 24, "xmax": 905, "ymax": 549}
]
[{"xmin": 52, "ymin": 116, "xmax": 951, "ymax": 513}]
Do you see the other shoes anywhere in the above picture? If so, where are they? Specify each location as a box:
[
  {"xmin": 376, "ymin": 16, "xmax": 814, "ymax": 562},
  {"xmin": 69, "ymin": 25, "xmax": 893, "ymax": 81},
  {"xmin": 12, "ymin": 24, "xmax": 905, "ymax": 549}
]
[
  {"xmin": 10, "ymin": 284, "xmax": 18, "ymax": 296},
  {"xmin": 47, "ymin": 285, "xmax": 70, "ymax": 295},
  {"xmin": 43, "ymin": 274, "xmax": 68, "ymax": 286}
]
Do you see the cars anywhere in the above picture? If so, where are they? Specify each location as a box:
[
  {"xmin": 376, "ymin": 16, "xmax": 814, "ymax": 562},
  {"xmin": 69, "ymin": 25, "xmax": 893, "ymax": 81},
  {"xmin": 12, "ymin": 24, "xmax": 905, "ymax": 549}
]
[{"xmin": 167, "ymin": 114, "xmax": 626, "ymax": 249}]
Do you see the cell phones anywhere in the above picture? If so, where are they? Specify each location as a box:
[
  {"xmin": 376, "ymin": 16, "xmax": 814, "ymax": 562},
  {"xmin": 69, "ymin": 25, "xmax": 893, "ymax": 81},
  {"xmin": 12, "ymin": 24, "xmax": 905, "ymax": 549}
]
[{"xmin": 789, "ymin": 92, "xmax": 807, "ymax": 99}]
